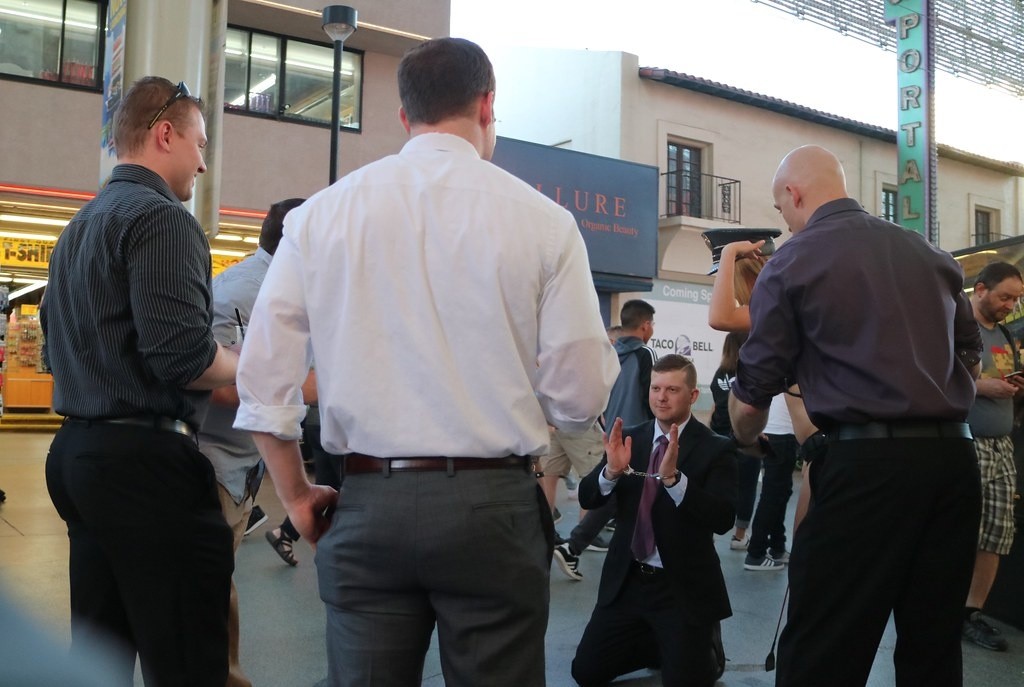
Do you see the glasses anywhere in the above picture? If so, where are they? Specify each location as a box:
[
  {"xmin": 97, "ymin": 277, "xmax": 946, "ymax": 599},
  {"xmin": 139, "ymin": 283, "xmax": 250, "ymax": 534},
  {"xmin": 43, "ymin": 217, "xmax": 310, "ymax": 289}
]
[{"xmin": 148, "ymin": 81, "xmax": 191, "ymax": 129}]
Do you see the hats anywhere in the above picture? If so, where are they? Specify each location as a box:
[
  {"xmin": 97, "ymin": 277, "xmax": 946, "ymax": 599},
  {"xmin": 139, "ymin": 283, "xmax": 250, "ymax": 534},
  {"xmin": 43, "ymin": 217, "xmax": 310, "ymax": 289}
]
[{"xmin": 700, "ymin": 228, "xmax": 782, "ymax": 277}]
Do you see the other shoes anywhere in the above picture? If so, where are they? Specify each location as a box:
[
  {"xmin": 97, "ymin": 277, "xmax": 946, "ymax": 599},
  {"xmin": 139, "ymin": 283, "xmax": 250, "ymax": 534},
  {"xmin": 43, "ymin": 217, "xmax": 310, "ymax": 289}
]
[
  {"xmin": 605, "ymin": 519, "xmax": 617, "ymax": 531},
  {"xmin": 244, "ymin": 505, "xmax": 269, "ymax": 536},
  {"xmin": 553, "ymin": 508, "xmax": 563, "ymax": 523}
]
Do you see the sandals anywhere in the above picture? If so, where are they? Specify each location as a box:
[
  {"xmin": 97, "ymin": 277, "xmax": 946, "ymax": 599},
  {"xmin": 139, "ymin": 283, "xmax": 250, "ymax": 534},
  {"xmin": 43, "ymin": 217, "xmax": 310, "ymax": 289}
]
[{"xmin": 265, "ymin": 528, "xmax": 298, "ymax": 565}]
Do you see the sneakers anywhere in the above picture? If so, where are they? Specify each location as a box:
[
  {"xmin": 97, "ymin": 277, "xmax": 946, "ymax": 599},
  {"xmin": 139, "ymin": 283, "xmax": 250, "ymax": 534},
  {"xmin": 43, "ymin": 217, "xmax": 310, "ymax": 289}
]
[
  {"xmin": 553, "ymin": 531, "xmax": 567, "ymax": 548},
  {"xmin": 730, "ymin": 534, "xmax": 750, "ymax": 550},
  {"xmin": 553, "ymin": 543, "xmax": 583, "ymax": 581},
  {"xmin": 744, "ymin": 551, "xmax": 791, "ymax": 571},
  {"xmin": 585, "ymin": 536, "xmax": 609, "ymax": 552},
  {"xmin": 962, "ymin": 612, "xmax": 1008, "ymax": 650}
]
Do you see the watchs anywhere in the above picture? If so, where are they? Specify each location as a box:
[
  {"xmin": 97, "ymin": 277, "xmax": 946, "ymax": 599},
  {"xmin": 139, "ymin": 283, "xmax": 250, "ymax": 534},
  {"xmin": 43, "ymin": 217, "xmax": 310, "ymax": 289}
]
[{"xmin": 665, "ymin": 470, "xmax": 681, "ymax": 488}]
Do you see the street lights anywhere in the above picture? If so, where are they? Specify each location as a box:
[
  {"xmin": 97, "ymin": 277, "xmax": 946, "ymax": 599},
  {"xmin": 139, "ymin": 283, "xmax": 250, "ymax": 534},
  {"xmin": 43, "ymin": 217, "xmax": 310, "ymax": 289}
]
[{"xmin": 321, "ymin": 5, "xmax": 359, "ymax": 185}]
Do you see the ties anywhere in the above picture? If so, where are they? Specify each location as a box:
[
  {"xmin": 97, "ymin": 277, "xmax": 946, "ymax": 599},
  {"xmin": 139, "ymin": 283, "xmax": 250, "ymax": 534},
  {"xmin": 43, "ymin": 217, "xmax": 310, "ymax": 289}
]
[{"xmin": 631, "ymin": 436, "xmax": 670, "ymax": 561}]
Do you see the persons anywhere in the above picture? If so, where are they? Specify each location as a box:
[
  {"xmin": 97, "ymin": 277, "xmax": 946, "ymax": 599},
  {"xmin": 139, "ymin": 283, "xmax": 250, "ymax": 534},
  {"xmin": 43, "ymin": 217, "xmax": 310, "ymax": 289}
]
[
  {"xmin": 244, "ymin": 500, "xmax": 269, "ymax": 536},
  {"xmin": 232, "ymin": 38, "xmax": 623, "ymax": 687},
  {"xmin": 960, "ymin": 261, "xmax": 1024, "ymax": 650},
  {"xmin": 701, "ymin": 228, "xmax": 819, "ymax": 572},
  {"xmin": 200, "ymin": 199, "xmax": 317, "ymax": 687},
  {"xmin": 0, "ymin": 489, "xmax": 6, "ymax": 502},
  {"xmin": 537, "ymin": 299, "xmax": 659, "ymax": 580},
  {"xmin": 728, "ymin": 146, "xmax": 984, "ymax": 687},
  {"xmin": 39, "ymin": 77, "xmax": 240, "ymax": 687},
  {"xmin": 570, "ymin": 355, "xmax": 740, "ymax": 687},
  {"xmin": 266, "ymin": 404, "xmax": 333, "ymax": 567}
]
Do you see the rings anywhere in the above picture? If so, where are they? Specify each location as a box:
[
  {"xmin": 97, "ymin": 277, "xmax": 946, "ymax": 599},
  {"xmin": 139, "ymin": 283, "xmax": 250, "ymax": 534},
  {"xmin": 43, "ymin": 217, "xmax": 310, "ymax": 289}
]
[{"xmin": 676, "ymin": 445, "xmax": 680, "ymax": 449}]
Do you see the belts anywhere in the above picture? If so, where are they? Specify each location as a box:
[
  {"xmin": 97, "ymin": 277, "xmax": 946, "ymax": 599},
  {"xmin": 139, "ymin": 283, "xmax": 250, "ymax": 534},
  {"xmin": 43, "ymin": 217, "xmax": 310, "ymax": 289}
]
[
  {"xmin": 346, "ymin": 450, "xmax": 531, "ymax": 478},
  {"xmin": 630, "ymin": 561, "xmax": 666, "ymax": 575},
  {"xmin": 829, "ymin": 423, "xmax": 973, "ymax": 440},
  {"xmin": 64, "ymin": 414, "xmax": 198, "ymax": 448}
]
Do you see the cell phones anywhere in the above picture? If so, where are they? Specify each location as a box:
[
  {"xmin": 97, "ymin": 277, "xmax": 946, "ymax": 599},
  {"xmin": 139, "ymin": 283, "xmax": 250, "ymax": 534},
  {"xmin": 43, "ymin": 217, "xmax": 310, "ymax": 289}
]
[{"xmin": 1004, "ymin": 371, "xmax": 1024, "ymax": 378}]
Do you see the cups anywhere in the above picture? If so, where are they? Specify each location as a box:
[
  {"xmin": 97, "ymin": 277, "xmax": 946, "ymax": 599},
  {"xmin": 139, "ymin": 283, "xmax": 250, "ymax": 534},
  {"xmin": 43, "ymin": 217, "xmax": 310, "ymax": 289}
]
[{"xmin": 237, "ymin": 326, "xmax": 248, "ymax": 344}]
